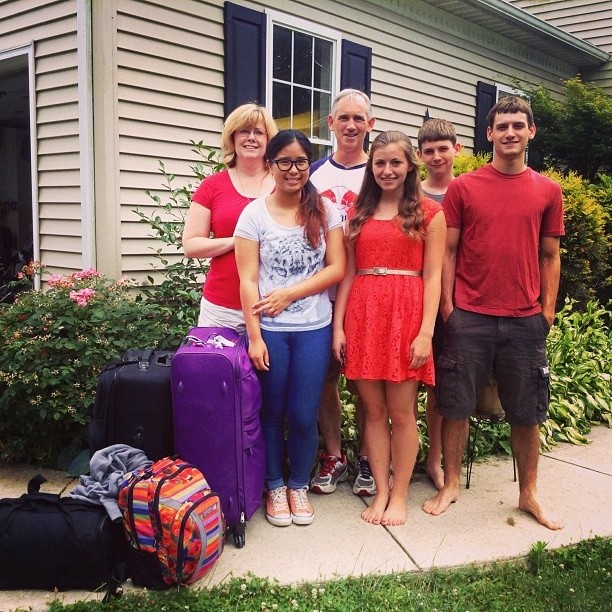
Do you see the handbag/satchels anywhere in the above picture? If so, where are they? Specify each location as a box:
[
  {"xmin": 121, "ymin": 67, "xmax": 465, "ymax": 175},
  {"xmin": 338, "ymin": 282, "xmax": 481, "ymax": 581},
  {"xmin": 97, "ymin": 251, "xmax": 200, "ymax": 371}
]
[{"xmin": 0, "ymin": 473, "xmax": 128, "ymax": 591}]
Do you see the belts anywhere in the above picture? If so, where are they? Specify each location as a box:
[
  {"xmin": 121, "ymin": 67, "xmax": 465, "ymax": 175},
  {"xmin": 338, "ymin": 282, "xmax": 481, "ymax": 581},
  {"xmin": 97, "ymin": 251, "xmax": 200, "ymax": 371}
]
[{"xmin": 357, "ymin": 267, "xmax": 422, "ymax": 278}]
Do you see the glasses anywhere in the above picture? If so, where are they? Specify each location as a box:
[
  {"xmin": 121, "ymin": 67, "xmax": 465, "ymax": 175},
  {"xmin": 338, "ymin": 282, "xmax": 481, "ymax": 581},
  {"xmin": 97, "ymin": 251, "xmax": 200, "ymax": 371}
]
[
  {"xmin": 267, "ymin": 158, "xmax": 312, "ymax": 171},
  {"xmin": 236, "ymin": 127, "xmax": 268, "ymax": 139}
]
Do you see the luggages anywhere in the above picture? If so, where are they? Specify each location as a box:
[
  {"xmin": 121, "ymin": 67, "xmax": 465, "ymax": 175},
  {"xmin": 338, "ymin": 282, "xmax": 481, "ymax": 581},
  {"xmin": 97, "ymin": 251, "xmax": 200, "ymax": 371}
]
[
  {"xmin": 88, "ymin": 346, "xmax": 174, "ymax": 464},
  {"xmin": 170, "ymin": 325, "xmax": 266, "ymax": 548}
]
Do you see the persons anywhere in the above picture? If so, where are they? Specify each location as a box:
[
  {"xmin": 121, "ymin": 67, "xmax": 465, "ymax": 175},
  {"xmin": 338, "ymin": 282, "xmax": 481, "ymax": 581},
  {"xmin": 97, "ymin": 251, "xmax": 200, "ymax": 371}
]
[
  {"xmin": 388, "ymin": 120, "xmax": 461, "ymax": 492},
  {"xmin": 332, "ymin": 131, "xmax": 448, "ymax": 527},
  {"xmin": 181, "ymin": 104, "xmax": 279, "ymax": 335},
  {"xmin": 308, "ymin": 89, "xmax": 377, "ymax": 496},
  {"xmin": 233, "ymin": 129, "xmax": 347, "ymax": 527},
  {"xmin": 421, "ymin": 96, "xmax": 566, "ymax": 530}
]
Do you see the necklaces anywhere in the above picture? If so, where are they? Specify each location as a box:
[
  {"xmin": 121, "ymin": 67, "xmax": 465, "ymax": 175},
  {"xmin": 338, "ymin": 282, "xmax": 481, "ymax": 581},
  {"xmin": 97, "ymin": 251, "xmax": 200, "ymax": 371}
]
[{"xmin": 235, "ymin": 164, "xmax": 266, "ymax": 204}]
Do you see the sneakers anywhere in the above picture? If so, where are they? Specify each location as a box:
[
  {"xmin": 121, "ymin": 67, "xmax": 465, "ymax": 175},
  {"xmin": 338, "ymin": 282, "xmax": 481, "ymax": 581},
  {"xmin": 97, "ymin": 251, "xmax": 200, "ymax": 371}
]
[
  {"xmin": 266, "ymin": 487, "xmax": 292, "ymax": 527},
  {"xmin": 287, "ymin": 489, "xmax": 315, "ymax": 525},
  {"xmin": 309, "ymin": 454, "xmax": 349, "ymax": 495},
  {"xmin": 352, "ymin": 454, "xmax": 394, "ymax": 497}
]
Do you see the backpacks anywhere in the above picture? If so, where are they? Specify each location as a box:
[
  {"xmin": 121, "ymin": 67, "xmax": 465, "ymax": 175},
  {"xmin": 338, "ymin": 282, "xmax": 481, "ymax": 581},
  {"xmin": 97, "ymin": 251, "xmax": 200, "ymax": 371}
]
[{"xmin": 117, "ymin": 457, "xmax": 226, "ymax": 590}]
